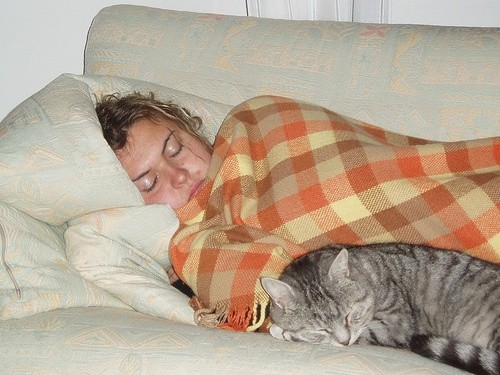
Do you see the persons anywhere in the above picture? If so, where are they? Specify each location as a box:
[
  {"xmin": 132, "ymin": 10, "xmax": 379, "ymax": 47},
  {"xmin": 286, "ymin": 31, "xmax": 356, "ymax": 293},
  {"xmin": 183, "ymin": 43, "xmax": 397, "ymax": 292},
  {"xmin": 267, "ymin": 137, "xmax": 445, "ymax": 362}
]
[{"xmin": 94, "ymin": 93, "xmax": 500, "ymax": 334}]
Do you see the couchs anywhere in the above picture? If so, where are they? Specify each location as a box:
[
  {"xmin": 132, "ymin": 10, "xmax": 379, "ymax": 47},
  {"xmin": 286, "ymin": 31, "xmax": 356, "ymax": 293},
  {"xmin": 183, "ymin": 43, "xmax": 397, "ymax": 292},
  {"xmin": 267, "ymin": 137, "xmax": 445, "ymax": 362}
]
[{"xmin": 0, "ymin": 5, "xmax": 500, "ymax": 375}]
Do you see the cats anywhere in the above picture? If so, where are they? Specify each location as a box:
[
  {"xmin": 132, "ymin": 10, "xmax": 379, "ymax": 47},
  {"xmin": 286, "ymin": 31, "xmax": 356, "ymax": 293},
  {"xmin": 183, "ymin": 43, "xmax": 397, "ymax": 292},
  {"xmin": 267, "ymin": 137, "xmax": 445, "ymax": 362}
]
[{"xmin": 260, "ymin": 241, "xmax": 500, "ymax": 375}]
